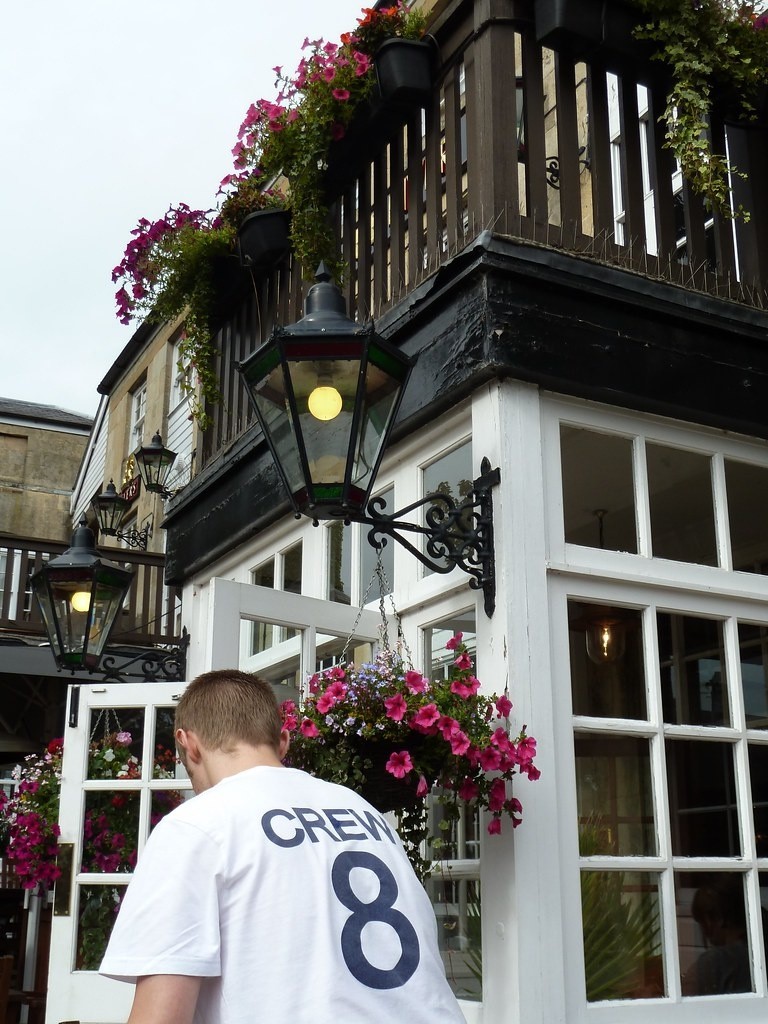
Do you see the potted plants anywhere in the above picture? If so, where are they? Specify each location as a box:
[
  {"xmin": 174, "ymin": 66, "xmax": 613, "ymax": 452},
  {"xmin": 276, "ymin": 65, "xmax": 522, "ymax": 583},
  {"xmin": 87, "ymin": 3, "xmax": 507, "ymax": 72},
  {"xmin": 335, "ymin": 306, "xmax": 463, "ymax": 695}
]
[
  {"xmin": 112, "ymin": 204, "xmax": 239, "ymax": 431},
  {"xmin": 630, "ymin": 0, "xmax": 768, "ymax": 223},
  {"xmin": 0, "ymin": 708, "xmax": 187, "ymax": 970},
  {"xmin": 211, "ymin": 166, "xmax": 294, "ymax": 271},
  {"xmin": 279, "ymin": 540, "xmax": 541, "ymax": 895},
  {"xmin": 340, "ymin": 0, "xmax": 444, "ymax": 109},
  {"xmin": 233, "ymin": 32, "xmax": 395, "ymax": 291}
]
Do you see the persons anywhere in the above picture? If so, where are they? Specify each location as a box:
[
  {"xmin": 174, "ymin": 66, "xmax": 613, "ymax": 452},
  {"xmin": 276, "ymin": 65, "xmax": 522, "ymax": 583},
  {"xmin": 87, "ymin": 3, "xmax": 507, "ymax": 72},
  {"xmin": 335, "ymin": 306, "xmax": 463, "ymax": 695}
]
[
  {"xmin": 97, "ymin": 668, "xmax": 469, "ymax": 1024},
  {"xmin": 678, "ymin": 878, "xmax": 767, "ymax": 996}
]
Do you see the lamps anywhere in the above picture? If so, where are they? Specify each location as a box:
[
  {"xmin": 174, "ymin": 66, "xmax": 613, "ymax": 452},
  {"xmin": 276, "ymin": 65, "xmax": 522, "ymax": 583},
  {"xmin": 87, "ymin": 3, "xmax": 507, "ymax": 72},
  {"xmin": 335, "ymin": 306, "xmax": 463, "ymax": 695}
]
[
  {"xmin": 585, "ymin": 507, "xmax": 624, "ymax": 668},
  {"xmin": 230, "ymin": 257, "xmax": 500, "ymax": 620},
  {"xmin": 133, "ymin": 429, "xmax": 179, "ymax": 499},
  {"xmin": 30, "ymin": 510, "xmax": 191, "ymax": 681},
  {"xmin": 91, "ymin": 478, "xmax": 149, "ymax": 550}
]
